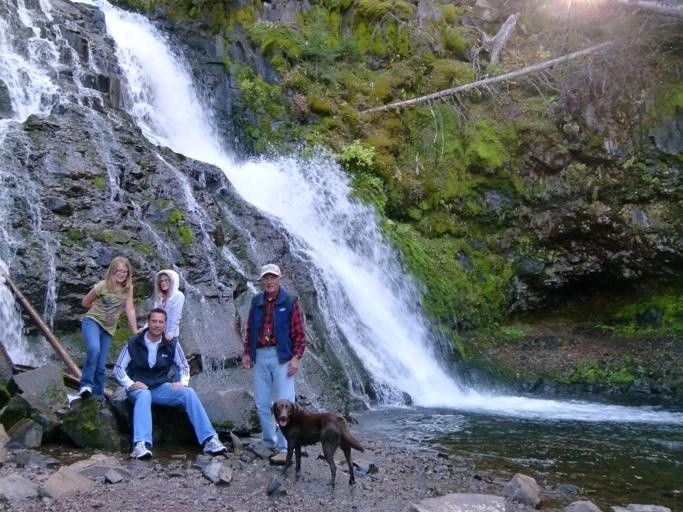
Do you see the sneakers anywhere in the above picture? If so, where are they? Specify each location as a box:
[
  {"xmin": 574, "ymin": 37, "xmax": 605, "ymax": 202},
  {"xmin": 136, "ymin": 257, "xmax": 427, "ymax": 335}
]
[
  {"xmin": 270, "ymin": 451, "xmax": 295, "ymax": 464},
  {"xmin": 204, "ymin": 434, "xmax": 227, "ymax": 455},
  {"xmin": 130, "ymin": 441, "xmax": 153, "ymax": 459},
  {"xmin": 79, "ymin": 384, "xmax": 93, "ymax": 397}
]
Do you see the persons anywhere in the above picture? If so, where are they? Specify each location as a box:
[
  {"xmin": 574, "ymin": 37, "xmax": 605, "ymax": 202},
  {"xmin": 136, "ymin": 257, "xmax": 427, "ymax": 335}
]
[
  {"xmin": 78, "ymin": 255, "xmax": 145, "ymax": 399},
  {"xmin": 111, "ymin": 307, "xmax": 228, "ymax": 459},
  {"xmin": 239, "ymin": 264, "xmax": 308, "ymax": 466},
  {"xmin": 135, "ymin": 268, "xmax": 191, "ymax": 388}
]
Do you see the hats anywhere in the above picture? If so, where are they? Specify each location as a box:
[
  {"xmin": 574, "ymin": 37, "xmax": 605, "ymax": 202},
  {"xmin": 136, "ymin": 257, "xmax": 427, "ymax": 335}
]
[{"xmin": 260, "ymin": 264, "xmax": 282, "ymax": 279}]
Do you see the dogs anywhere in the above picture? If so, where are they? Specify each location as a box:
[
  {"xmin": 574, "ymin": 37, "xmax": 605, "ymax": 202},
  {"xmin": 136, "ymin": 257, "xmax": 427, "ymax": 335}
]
[{"xmin": 270, "ymin": 399, "xmax": 364, "ymax": 488}]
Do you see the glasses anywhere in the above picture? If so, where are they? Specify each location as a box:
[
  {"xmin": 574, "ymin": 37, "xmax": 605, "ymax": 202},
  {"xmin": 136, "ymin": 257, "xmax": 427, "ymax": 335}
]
[{"xmin": 160, "ymin": 279, "xmax": 170, "ymax": 283}]
